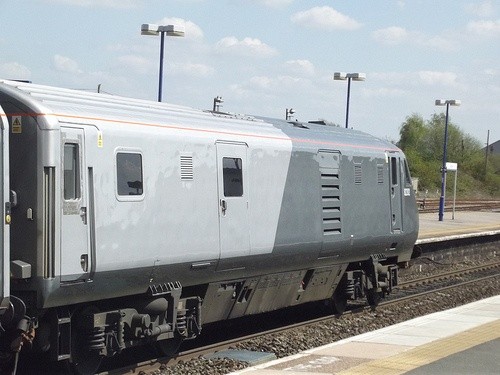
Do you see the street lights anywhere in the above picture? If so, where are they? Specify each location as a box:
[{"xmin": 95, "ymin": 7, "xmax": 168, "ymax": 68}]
[
  {"xmin": 435, "ymin": 99, "xmax": 462, "ymax": 220},
  {"xmin": 333, "ymin": 72, "xmax": 366, "ymax": 130},
  {"xmin": 140, "ymin": 23, "xmax": 185, "ymax": 103}
]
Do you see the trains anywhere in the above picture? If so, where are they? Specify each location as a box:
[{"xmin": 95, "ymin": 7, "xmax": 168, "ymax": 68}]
[{"xmin": 0, "ymin": 78, "xmax": 419, "ymax": 375}]
